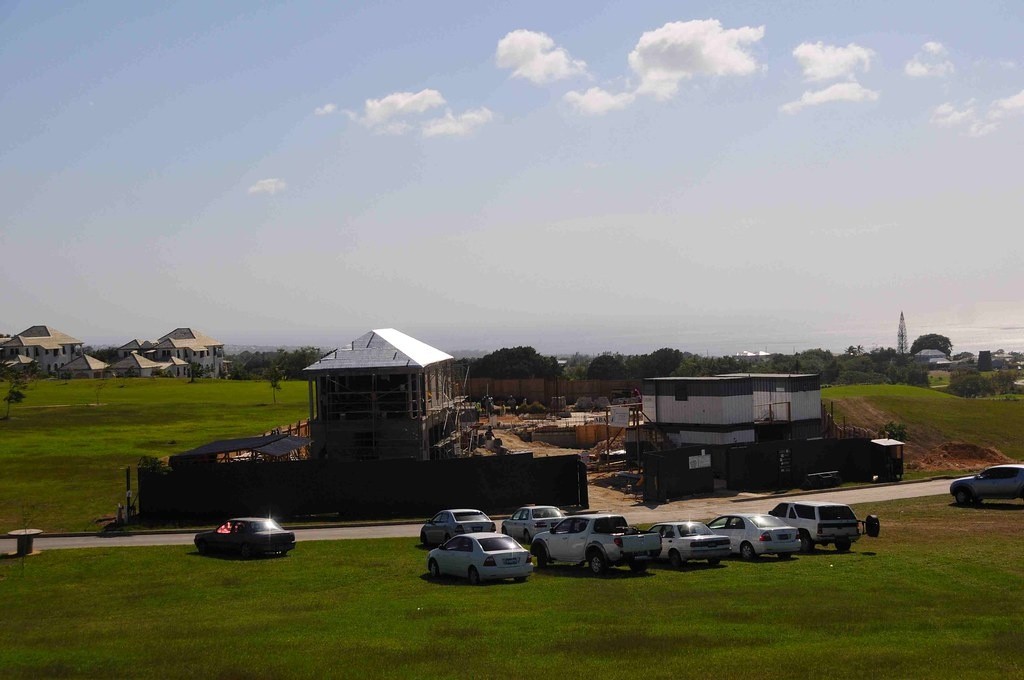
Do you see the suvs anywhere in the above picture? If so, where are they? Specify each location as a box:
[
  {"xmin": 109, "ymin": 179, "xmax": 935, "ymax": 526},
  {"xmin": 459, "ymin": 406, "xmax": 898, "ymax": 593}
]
[
  {"xmin": 949, "ymin": 465, "xmax": 1024, "ymax": 506},
  {"xmin": 768, "ymin": 501, "xmax": 880, "ymax": 553}
]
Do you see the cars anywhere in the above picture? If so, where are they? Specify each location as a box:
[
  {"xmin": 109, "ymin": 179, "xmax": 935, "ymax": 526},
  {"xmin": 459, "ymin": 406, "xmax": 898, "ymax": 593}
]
[
  {"xmin": 501, "ymin": 506, "xmax": 566, "ymax": 545},
  {"xmin": 420, "ymin": 509, "xmax": 496, "ymax": 547},
  {"xmin": 646, "ymin": 520, "xmax": 731, "ymax": 568},
  {"xmin": 194, "ymin": 517, "xmax": 296, "ymax": 560},
  {"xmin": 706, "ymin": 513, "xmax": 802, "ymax": 561},
  {"xmin": 427, "ymin": 532, "xmax": 534, "ymax": 586}
]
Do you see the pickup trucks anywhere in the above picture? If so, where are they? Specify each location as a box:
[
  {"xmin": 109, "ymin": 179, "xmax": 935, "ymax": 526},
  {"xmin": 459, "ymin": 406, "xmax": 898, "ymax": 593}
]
[{"xmin": 529, "ymin": 513, "xmax": 663, "ymax": 574}]
